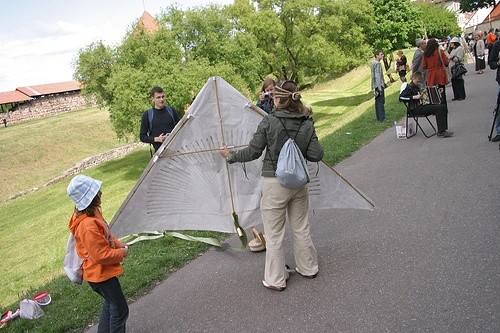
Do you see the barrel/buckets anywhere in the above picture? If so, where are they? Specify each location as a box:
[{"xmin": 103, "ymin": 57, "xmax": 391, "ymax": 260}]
[{"xmin": 34, "ymin": 292, "xmax": 52, "ymax": 306}]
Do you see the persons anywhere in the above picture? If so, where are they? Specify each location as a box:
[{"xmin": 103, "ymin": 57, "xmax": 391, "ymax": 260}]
[
  {"xmin": 396, "ymin": 50, "xmax": 407, "ymax": 81},
  {"xmin": 422, "ymin": 38, "xmax": 449, "ymax": 112},
  {"xmin": 255, "ymin": 78, "xmax": 278, "ymax": 113},
  {"xmin": 411, "ymin": 38, "xmax": 427, "ymax": 89},
  {"xmin": 398, "ymin": 70, "xmax": 455, "ymax": 137},
  {"xmin": 473, "ymin": 33, "xmax": 486, "ymax": 74},
  {"xmin": 66, "ymin": 173, "xmax": 129, "ymax": 332},
  {"xmin": 489, "ymin": 37, "xmax": 500, "ymax": 142},
  {"xmin": 449, "ymin": 36, "xmax": 466, "ymax": 100},
  {"xmin": 221, "ymin": 79, "xmax": 325, "ymax": 291},
  {"xmin": 140, "ymin": 86, "xmax": 181, "ymax": 151},
  {"xmin": 486, "ymin": 27, "xmax": 496, "ymax": 64},
  {"xmin": 370, "ymin": 50, "xmax": 391, "ymax": 122}
]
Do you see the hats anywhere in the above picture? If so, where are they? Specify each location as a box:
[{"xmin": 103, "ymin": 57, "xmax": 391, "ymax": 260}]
[
  {"xmin": 67, "ymin": 173, "xmax": 104, "ymax": 211},
  {"xmin": 450, "ymin": 37, "xmax": 459, "ymax": 42}
]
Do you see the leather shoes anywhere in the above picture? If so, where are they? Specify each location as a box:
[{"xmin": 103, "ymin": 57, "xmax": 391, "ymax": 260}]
[{"xmin": 437, "ymin": 131, "xmax": 455, "ymax": 136}]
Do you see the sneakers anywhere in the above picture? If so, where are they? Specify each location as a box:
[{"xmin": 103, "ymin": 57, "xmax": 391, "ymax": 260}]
[{"xmin": 262, "ymin": 279, "xmax": 285, "ymax": 290}]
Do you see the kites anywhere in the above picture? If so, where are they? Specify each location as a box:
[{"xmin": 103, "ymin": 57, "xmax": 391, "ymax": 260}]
[{"xmin": 108, "ymin": 76, "xmax": 377, "ymax": 238}]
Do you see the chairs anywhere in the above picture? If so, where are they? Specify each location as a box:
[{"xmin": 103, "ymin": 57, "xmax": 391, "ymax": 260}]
[{"xmin": 400, "ymin": 84, "xmax": 437, "ymax": 139}]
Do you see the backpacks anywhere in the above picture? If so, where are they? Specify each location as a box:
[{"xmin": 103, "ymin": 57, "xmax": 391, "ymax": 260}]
[{"xmin": 265, "ymin": 114, "xmax": 312, "ymax": 188}]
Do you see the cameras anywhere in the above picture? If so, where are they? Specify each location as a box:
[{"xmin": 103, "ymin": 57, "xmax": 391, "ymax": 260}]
[{"xmin": 263, "ymin": 93, "xmax": 270, "ymax": 100}]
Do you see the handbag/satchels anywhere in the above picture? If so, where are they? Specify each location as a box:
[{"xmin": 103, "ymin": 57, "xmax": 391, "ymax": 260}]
[
  {"xmin": 450, "ymin": 56, "xmax": 467, "ymax": 79},
  {"xmin": 62, "ymin": 218, "xmax": 111, "ymax": 285},
  {"xmin": 444, "ymin": 66, "xmax": 450, "ymax": 83},
  {"xmin": 17, "ymin": 289, "xmax": 45, "ymax": 319}
]
